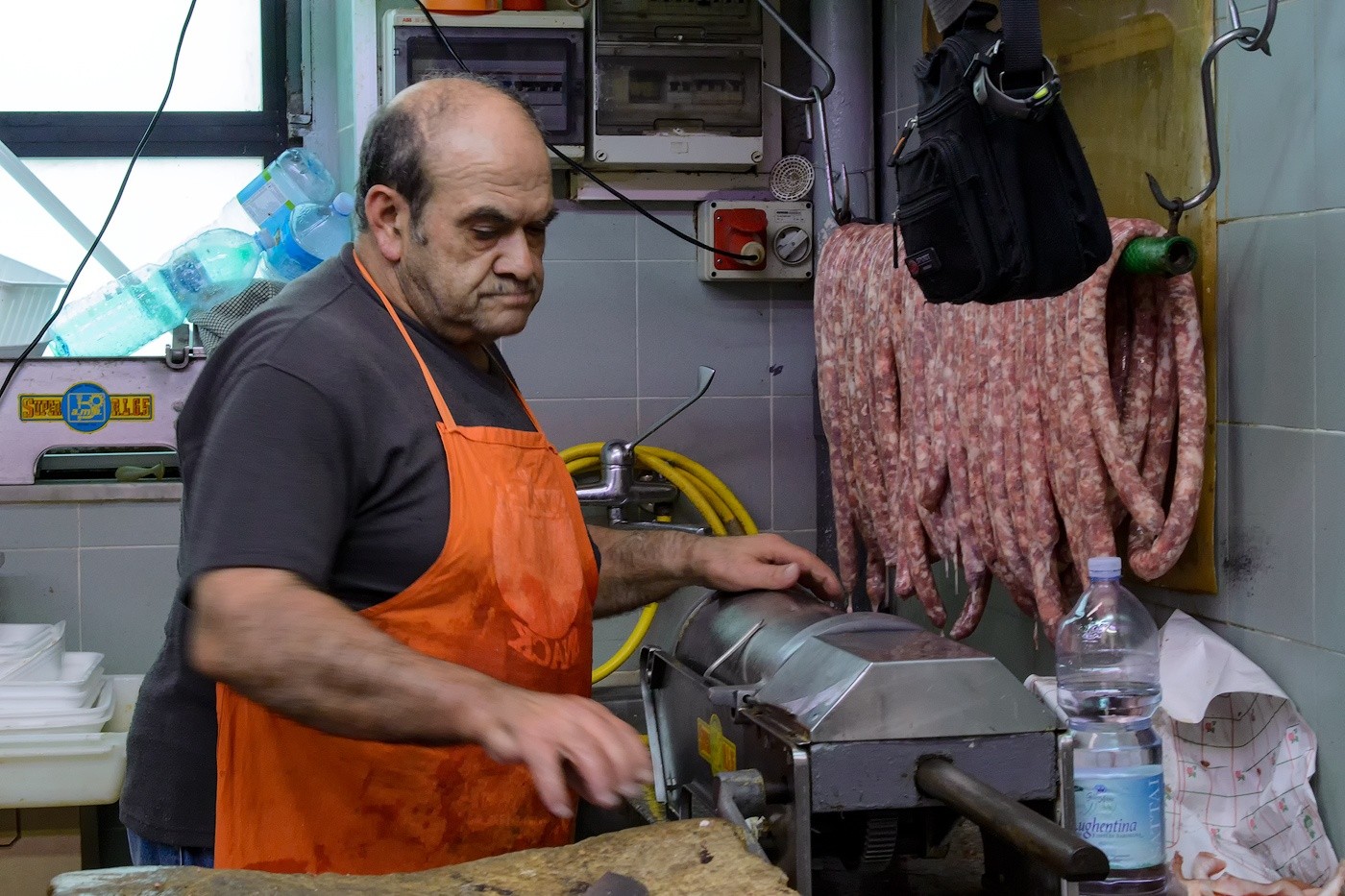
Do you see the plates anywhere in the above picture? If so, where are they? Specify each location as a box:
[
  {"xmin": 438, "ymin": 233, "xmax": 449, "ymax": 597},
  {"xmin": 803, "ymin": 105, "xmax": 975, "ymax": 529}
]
[{"xmin": 0, "ymin": 620, "xmax": 114, "ymax": 731}]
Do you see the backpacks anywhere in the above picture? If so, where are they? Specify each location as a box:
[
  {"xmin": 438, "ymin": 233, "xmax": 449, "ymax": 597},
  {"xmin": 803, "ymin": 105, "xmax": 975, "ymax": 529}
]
[{"xmin": 886, "ymin": 2, "xmax": 1116, "ymax": 305}]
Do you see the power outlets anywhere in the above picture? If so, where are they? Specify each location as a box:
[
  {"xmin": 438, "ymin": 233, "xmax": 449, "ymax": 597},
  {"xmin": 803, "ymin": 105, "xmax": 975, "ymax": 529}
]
[{"xmin": 696, "ymin": 200, "xmax": 812, "ymax": 284}]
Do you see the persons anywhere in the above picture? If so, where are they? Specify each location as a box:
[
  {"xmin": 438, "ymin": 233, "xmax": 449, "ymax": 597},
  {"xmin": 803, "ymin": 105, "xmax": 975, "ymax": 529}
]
[{"xmin": 118, "ymin": 74, "xmax": 846, "ymax": 878}]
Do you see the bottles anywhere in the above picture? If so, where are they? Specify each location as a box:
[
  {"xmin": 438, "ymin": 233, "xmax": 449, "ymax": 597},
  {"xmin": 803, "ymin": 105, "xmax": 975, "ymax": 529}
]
[
  {"xmin": 43, "ymin": 228, "xmax": 276, "ymax": 357},
  {"xmin": 1055, "ymin": 557, "xmax": 1166, "ymax": 895},
  {"xmin": 255, "ymin": 191, "xmax": 357, "ymax": 281},
  {"xmin": 190, "ymin": 146, "xmax": 336, "ymax": 250}
]
